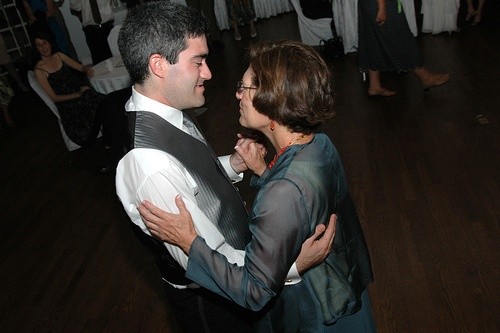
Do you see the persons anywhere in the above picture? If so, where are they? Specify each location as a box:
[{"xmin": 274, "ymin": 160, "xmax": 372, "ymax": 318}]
[
  {"xmin": 0, "ymin": 0, "xmax": 130, "ymax": 174},
  {"xmin": 136, "ymin": 39, "xmax": 376, "ymax": 333},
  {"xmin": 186, "ymin": 0, "xmax": 486, "ymax": 97},
  {"xmin": 111, "ymin": 0, "xmax": 338, "ymax": 333}
]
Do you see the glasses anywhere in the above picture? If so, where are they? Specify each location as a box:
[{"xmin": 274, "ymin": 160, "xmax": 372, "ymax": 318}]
[{"xmin": 236, "ymin": 81, "xmax": 258, "ymax": 91}]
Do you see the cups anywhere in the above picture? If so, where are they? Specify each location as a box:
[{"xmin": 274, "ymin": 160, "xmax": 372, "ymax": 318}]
[{"xmin": 104, "ymin": 61, "xmax": 114, "ymax": 71}]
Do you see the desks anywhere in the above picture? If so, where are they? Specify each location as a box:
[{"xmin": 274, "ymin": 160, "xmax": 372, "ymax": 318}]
[{"xmin": 86, "ymin": 55, "xmax": 134, "ymax": 94}]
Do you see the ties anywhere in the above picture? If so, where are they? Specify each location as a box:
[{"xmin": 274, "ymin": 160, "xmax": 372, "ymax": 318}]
[
  {"xmin": 183, "ymin": 112, "xmax": 207, "ymax": 144},
  {"xmin": 89, "ymin": 0, "xmax": 102, "ymax": 24}
]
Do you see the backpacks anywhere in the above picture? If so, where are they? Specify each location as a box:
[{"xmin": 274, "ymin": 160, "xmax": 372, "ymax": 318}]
[{"xmin": 320, "ymin": 35, "xmax": 345, "ymax": 63}]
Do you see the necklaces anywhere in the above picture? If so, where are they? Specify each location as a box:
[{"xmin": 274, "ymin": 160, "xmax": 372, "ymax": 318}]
[{"xmin": 267, "ymin": 133, "xmax": 307, "ymax": 169}]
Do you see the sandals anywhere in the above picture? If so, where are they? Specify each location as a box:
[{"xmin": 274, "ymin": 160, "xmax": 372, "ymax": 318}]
[
  {"xmin": 367, "ymin": 88, "xmax": 396, "ymax": 98},
  {"xmin": 423, "ymin": 73, "xmax": 449, "ymax": 91}
]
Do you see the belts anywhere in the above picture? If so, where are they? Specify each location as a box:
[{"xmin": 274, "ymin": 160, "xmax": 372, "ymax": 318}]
[{"xmin": 83, "ymin": 20, "xmax": 112, "ymax": 28}]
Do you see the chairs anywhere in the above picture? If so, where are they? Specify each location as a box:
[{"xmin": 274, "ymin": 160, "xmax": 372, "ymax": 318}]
[
  {"xmin": 27, "ymin": 70, "xmax": 103, "ymax": 152},
  {"xmin": 291, "ymin": 0, "xmax": 334, "ymax": 46}
]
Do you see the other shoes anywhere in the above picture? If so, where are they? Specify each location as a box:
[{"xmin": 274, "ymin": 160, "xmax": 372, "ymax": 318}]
[
  {"xmin": 251, "ymin": 32, "xmax": 256, "ymax": 36},
  {"xmin": 234, "ymin": 36, "xmax": 242, "ymax": 40},
  {"xmin": 96, "ymin": 158, "xmax": 111, "ymax": 172}
]
